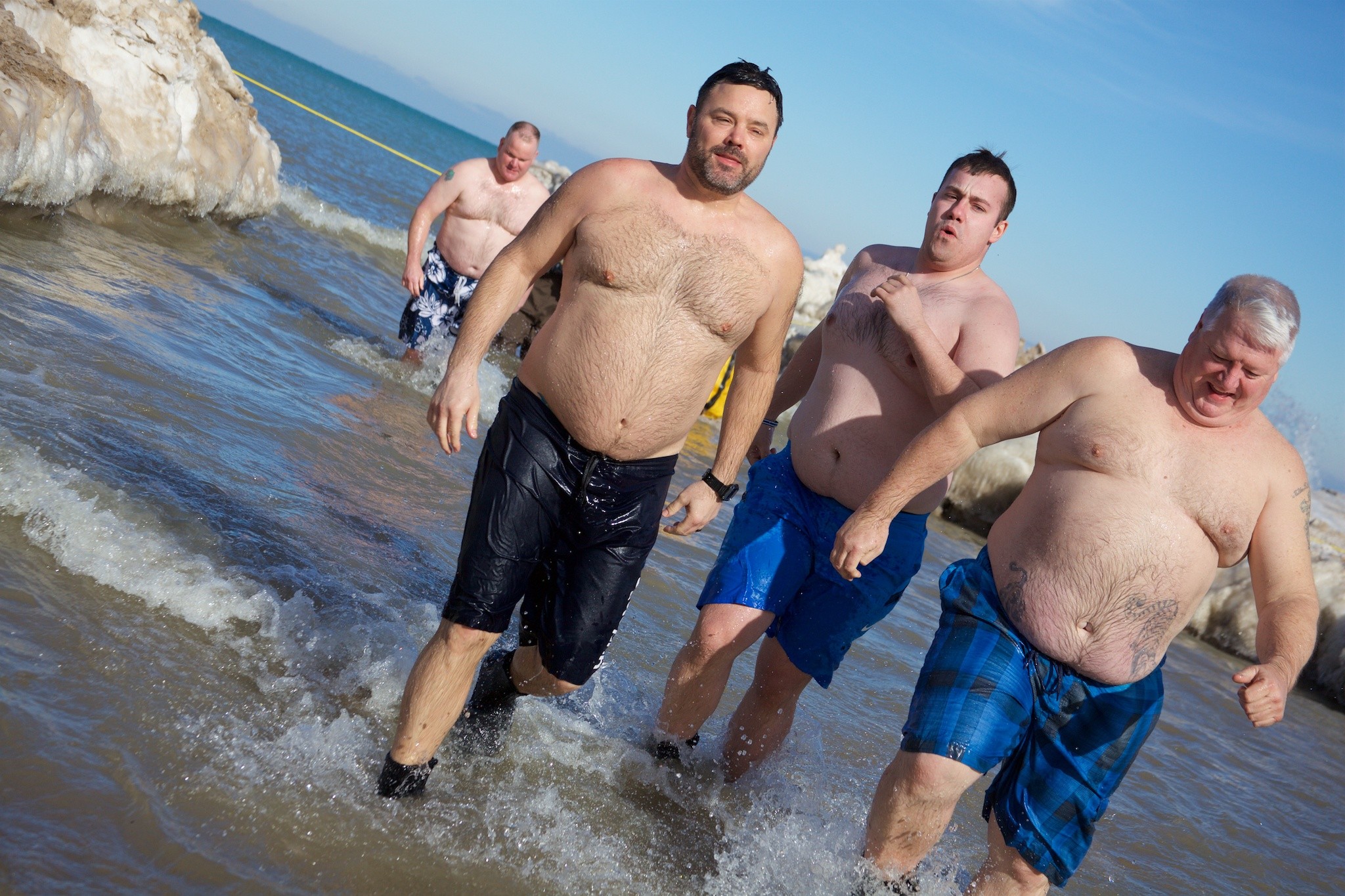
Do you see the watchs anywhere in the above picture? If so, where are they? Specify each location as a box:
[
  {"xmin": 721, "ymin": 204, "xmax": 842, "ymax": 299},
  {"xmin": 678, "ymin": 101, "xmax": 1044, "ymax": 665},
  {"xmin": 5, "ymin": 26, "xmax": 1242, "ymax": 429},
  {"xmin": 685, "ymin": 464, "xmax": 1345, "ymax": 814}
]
[{"xmin": 701, "ymin": 467, "xmax": 739, "ymax": 501}]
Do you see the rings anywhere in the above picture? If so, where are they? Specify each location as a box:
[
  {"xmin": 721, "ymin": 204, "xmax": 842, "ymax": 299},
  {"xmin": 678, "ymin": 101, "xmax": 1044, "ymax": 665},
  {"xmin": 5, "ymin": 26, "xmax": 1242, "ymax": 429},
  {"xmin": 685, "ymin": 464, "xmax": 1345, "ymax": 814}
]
[{"xmin": 696, "ymin": 529, "xmax": 702, "ymax": 532}]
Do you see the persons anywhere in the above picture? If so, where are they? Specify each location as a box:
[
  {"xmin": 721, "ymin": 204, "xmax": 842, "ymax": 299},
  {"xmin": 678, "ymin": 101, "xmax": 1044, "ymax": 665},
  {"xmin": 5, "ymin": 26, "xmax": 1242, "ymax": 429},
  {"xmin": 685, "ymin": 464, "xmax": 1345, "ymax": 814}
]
[
  {"xmin": 828, "ymin": 274, "xmax": 1319, "ymax": 896},
  {"xmin": 402, "ymin": 122, "xmax": 551, "ymax": 366},
  {"xmin": 647, "ymin": 145, "xmax": 1016, "ymax": 786},
  {"xmin": 377, "ymin": 56, "xmax": 804, "ymax": 802}
]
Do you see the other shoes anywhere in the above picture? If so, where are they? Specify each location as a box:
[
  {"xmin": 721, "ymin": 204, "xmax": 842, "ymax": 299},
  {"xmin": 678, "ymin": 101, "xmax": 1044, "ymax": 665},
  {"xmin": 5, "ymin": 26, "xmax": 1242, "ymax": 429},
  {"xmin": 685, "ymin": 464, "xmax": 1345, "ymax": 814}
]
[
  {"xmin": 376, "ymin": 751, "xmax": 439, "ymax": 799},
  {"xmin": 463, "ymin": 650, "xmax": 520, "ymax": 758}
]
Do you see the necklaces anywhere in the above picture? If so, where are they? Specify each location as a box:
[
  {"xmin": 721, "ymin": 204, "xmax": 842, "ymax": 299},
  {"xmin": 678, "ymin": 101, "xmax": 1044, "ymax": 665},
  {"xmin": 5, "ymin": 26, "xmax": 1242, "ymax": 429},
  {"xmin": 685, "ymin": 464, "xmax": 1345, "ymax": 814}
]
[{"xmin": 905, "ymin": 259, "xmax": 980, "ymax": 293}]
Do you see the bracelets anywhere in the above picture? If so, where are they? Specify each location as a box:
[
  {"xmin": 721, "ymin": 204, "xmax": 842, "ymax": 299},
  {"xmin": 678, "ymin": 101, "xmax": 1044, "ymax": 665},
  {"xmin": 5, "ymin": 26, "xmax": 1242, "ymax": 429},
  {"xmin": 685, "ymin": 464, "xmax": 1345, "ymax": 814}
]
[{"xmin": 762, "ymin": 418, "xmax": 779, "ymax": 427}]
